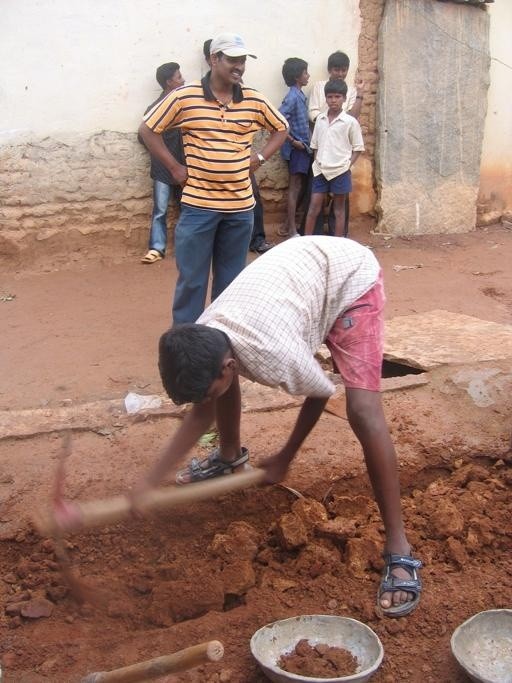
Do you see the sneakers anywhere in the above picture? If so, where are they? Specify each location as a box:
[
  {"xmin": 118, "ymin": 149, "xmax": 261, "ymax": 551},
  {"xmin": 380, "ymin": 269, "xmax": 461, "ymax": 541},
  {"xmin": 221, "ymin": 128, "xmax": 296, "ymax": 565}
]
[{"xmin": 256, "ymin": 240, "xmax": 273, "ymax": 252}]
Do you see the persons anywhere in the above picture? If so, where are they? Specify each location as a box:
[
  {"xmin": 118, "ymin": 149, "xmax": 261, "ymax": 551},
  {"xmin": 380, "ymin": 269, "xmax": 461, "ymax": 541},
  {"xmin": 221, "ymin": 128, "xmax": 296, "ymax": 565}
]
[
  {"xmin": 297, "ymin": 50, "xmax": 364, "ymax": 240},
  {"xmin": 305, "ymin": 77, "xmax": 365, "ymax": 237},
  {"xmin": 127, "ymin": 234, "xmax": 423, "ymax": 618},
  {"xmin": 202, "ymin": 38, "xmax": 270, "ymax": 252},
  {"xmin": 138, "ymin": 61, "xmax": 186, "ymax": 264},
  {"xmin": 277, "ymin": 56, "xmax": 311, "ymax": 237},
  {"xmin": 137, "ymin": 35, "xmax": 291, "ymax": 328}
]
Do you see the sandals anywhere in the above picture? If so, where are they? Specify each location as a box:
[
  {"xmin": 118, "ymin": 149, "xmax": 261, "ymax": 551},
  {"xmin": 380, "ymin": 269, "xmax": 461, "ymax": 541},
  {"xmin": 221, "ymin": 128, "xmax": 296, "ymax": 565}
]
[
  {"xmin": 141, "ymin": 250, "xmax": 163, "ymax": 263},
  {"xmin": 376, "ymin": 555, "xmax": 422, "ymax": 619},
  {"xmin": 175, "ymin": 446, "xmax": 248, "ymax": 487}
]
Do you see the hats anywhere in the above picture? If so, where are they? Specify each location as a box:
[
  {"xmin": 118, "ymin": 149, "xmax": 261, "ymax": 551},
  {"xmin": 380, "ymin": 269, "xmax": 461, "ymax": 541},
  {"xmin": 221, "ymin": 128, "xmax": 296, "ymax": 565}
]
[{"xmin": 209, "ymin": 32, "xmax": 257, "ymax": 58}]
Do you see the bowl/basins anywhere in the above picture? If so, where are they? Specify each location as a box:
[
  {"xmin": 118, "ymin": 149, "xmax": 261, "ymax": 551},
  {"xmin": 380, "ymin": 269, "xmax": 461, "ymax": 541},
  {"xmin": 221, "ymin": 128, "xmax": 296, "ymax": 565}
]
[
  {"xmin": 250, "ymin": 614, "xmax": 385, "ymax": 682},
  {"xmin": 450, "ymin": 608, "xmax": 512, "ymax": 683}
]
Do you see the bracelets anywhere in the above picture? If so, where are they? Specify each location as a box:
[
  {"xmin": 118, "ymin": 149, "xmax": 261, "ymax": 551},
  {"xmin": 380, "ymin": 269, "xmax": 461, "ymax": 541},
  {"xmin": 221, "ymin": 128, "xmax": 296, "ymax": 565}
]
[{"xmin": 355, "ymin": 95, "xmax": 363, "ymax": 99}]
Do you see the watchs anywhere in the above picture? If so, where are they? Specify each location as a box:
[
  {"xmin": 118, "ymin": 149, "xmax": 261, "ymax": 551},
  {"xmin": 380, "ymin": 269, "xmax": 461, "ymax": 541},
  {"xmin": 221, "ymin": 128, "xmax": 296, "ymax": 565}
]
[{"xmin": 257, "ymin": 152, "xmax": 266, "ymax": 166}]
